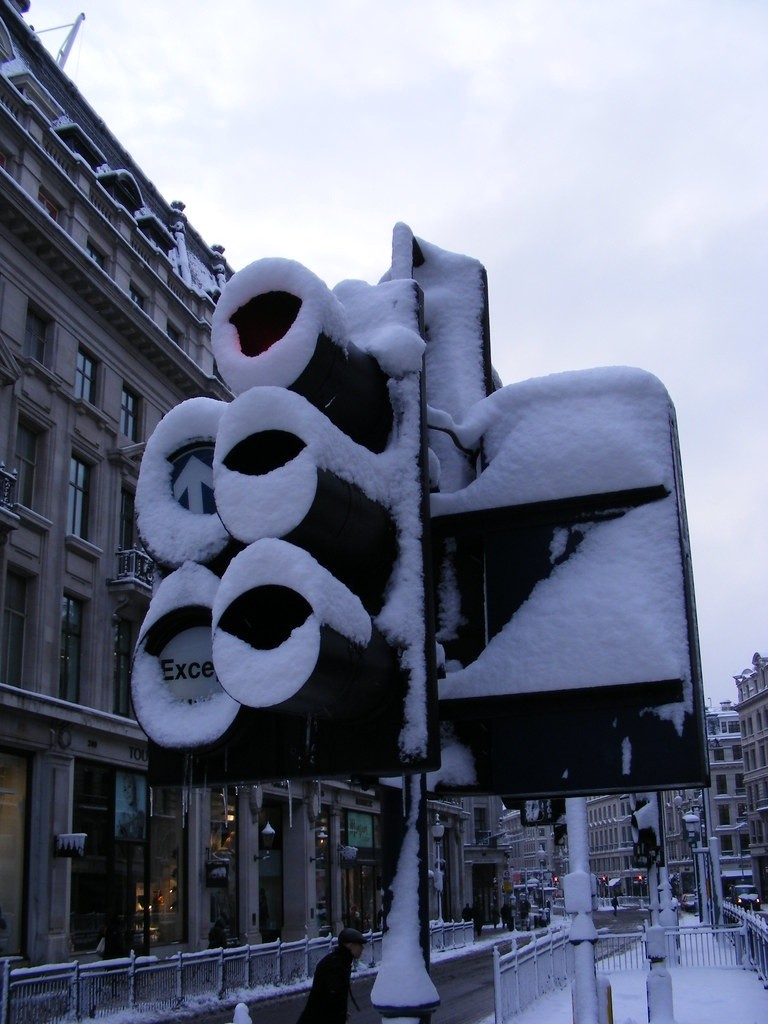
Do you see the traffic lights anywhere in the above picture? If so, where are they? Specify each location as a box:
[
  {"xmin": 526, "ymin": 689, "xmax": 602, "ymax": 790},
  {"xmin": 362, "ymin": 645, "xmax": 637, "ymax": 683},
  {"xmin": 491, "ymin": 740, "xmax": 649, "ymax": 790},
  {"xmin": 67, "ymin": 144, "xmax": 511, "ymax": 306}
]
[
  {"xmin": 602, "ymin": 876, "xmax": 607, "ymax": 881},
  {"xmin": 129, "ymin": 393, "xmax": 288, "ymax": 790},
  {"xmin": 210, "ymin": 256, "xmax": 441, "ymax": 779},
  {"xmin": 554, "ymin": 877, "xmax": 558, "ymax": 882},
  {"xmin": 638, "ymin": 875, "xmax": 642, "ymax": 880},
  {"xmin": 627, "ymin": 792, "xmax": 664, "ymax": 869}
]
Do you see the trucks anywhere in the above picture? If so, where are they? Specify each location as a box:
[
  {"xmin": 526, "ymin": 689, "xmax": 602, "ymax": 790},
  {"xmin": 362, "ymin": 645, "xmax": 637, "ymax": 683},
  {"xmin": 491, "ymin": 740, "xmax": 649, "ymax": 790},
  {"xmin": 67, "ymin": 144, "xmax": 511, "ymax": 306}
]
[{"xmin": 734, "ymin": 884, "xmax": 760, "ymax": 911}]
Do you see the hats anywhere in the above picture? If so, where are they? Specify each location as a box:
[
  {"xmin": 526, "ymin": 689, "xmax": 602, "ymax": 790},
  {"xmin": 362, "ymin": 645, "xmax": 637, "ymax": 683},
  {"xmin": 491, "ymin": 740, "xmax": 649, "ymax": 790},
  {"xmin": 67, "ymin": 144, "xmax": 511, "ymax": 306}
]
[{"xmin": 338, "ymin": 928, "xmax": 370, "ymax": 946}]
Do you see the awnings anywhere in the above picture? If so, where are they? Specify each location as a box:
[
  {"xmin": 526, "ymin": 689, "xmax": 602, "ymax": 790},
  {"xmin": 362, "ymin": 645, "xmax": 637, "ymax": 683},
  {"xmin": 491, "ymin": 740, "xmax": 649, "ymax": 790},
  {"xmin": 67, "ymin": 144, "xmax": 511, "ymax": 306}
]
[
  {"xmin": 721, "ymin": 870, "xmax": 753, "ymax": 877},
  {"xmin": 608, "ymin": 878, "xmax": 623, "ymax": 887},
  {"xmin": 514, "ymin": 884, "xmax": 557, "ymax": 891}
]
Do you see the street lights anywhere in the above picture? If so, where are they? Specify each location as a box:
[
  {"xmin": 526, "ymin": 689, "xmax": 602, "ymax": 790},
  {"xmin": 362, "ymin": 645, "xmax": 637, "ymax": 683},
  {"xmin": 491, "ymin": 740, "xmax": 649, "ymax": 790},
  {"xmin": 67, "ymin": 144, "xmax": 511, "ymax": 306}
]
[
  {"xmin": 430, "ymin": 812, "xmax": 446, "ymax": 956},
  {"xmin": 674, "ymin": 794, "xmax": 705, "ymax": 927},
  {"xmin": 536, "ymin": 844, "xmax": 548, "ymax": 928}
]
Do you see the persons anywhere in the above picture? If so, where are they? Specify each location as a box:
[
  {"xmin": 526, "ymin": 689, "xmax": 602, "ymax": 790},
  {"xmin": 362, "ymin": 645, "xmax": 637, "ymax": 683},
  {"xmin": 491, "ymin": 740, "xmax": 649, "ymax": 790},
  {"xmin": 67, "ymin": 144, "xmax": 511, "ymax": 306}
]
[
  {"xmin": 348, "ymin": 904, "xmax": 362, "ymax": 933},
  {"xmin": 462, "ymin": 898, "xmax": 552, "ymax": 937},
  {"xmin": 261, "ymin": 920, "xmax": 280, "ymax": 943},
  {"xmin": 93, "ymin": 922, "xmax": 153, "ymax": 999},
  {"xmin": 297, "ymin": 928, "xmax": 368, "ymax": 1024},
  {"xmin": 611, "ymin": 895, "xmax": 620, "ymax": 915},
  {"xmin": 208, "ymin": 918, "xmax": 228, "ymax": 982}
]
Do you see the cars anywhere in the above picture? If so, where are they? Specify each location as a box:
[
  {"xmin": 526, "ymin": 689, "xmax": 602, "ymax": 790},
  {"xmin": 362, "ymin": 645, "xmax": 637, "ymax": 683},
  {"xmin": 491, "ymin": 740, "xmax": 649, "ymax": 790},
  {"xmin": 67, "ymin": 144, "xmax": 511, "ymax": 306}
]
[{"xmin": 681, "ymin": 894, "xmax": 695, "ymax": 911}]
